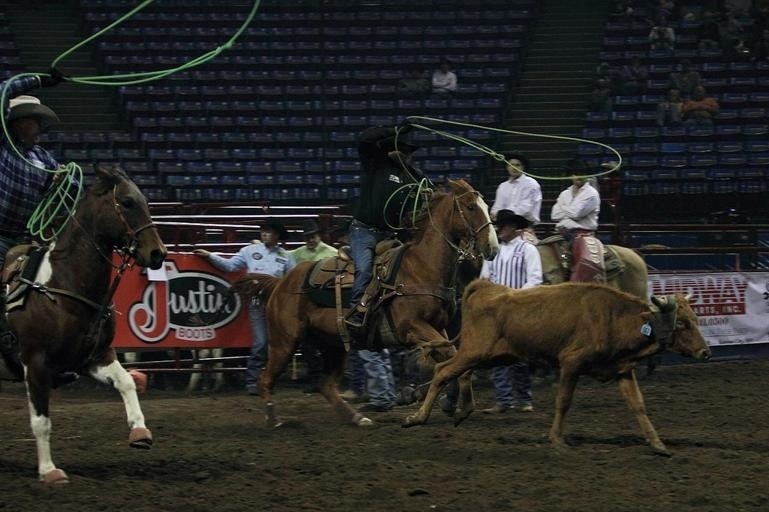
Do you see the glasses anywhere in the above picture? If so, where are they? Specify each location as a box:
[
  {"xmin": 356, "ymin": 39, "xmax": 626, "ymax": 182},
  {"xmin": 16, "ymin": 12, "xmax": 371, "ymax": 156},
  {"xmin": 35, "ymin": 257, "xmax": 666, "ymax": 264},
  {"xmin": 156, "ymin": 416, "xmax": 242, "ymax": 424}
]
[{"xmin": 26, "ymin": 115, "xmax": 49, "ymax": 130}]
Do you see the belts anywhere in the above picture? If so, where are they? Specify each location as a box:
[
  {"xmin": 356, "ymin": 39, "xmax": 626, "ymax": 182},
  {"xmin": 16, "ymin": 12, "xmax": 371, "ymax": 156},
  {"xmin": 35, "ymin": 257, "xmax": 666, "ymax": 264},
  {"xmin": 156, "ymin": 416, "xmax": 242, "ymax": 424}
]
[{"xmin": 351, "ymin": 225, "xmax": 398, "ymax": 238}]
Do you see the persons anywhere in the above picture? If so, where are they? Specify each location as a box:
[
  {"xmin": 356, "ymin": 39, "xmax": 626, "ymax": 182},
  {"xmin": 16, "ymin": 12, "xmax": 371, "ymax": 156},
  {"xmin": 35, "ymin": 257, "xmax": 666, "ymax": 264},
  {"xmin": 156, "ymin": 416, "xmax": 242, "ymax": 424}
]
[
  {"xmin": 251, "ymin": 217, "xmax": 338, "ymax": 394},
  {"xmin": 550, "ymin": 160, "xmax": 601, "ymax": 256},
  {"xmin": 432, "ymin": 60, "xmax": 458, "ymax": 94},
  {"xmin": 343, "ymin": 122, "xmax": 422, "ymax": 315},
  {"xmin": 482, "ymin": 210, "xmax": 543, "ymax": 416},
  {"xmin": 194, "ymin": 219, "xmax": 297, "ymax": 394},
  {"xmin": 589, "ymin": 0, "xmax": 743, "ymax": 125},
  {"xmin": 0, "ymin": 68, "xmax": 69, "ymax": 349},
  {"xmin": 489, "ymin": 152, "xmax": 543, "ymax": 245},
  {"xmin": 337, "ymin": 342, "xmax": 396, "ymax": 411}
]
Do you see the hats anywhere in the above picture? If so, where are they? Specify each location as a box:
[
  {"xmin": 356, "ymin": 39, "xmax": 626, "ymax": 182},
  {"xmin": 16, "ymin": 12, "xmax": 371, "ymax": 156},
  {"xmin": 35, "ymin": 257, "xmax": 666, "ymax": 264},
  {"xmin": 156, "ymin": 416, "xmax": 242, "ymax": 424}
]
[
  {"xmin": 503, "ymin": 150, "xmax": 530, "ymax": 176},
  {"xmin": 301, "ymin": 220, "xmax": 320, "ymax": 236},
  {"xmin": 489, "ymin": 209, "xmax": 536, "ymax": 229},
  {"xmin": 5, "ymin": 95, "xmax": 62, "ymax": 135},
  {"xmin": 379, "ymin": 133, "xmax": 420, "ymax": 155},
  {"xmin": 560, "ymin": 157, "xmax": 595, "ymax": 174},
  {"xmin": 252, "ymin": 219, "xmax": 284, "ymax": 234}
]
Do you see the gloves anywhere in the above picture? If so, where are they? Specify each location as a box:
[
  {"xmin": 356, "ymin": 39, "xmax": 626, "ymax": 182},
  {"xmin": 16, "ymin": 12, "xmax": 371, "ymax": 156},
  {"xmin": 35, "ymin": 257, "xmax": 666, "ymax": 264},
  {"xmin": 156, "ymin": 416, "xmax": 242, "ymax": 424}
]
[
  {"xmin": 396, "ymin": 118, "xmax": 417, "ymax": 134},
  {"xmin": 37, "ymin": 66, "xmax": 74, "ymax": 89}
]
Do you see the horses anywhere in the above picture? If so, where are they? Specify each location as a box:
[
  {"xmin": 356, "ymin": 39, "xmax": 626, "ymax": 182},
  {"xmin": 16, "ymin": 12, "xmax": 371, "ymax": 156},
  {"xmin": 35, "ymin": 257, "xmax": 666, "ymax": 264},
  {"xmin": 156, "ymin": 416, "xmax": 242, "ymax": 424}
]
[
  {"xmin": 0, "ymin": 161, "xmax": 166, "ymax": 486},
  {"xmin": 401, "ymin": 278, "xmax": 711, "ymax": 454},
  {"xmin": 225, "ymin": 180, "xmax": 499, "ymax": 429},
  {"xmin": 455, "ymin": 242, "xmax": 647, "ymax": 301}
]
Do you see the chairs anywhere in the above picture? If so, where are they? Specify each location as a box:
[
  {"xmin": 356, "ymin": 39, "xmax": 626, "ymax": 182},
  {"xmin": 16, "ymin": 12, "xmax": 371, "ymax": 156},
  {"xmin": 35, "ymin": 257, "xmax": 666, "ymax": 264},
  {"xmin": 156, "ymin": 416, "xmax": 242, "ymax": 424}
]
[{"xmin": 572, "ymin": 0, "xmax": 769, "ymax": 194}]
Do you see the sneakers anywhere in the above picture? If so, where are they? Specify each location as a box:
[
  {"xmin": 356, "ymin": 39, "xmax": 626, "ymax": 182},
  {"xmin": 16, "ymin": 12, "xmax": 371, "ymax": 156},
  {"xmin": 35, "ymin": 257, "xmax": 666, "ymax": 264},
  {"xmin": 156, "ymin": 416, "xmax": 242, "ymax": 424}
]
[
  {"xmin": 51, "ymin": 373, "xmax": 79, "ymax": 389},
  {"xmin": 337, "ymin": 376, "xmax": 394, "ymax": 412},
  {"xmin": 246, "ymin": 382, "xmax": 261, "ymax": 396},
  {"xmin": 480, "ymin": 400, "xmax": 534, "ymax": 414},
  {"xmin": 349, "ymin": 313, "xmax": 363, "ymax": 324},
  {"xmin": 303, "ymin": 381, "xmax": 319, "ymax": 393}
]
